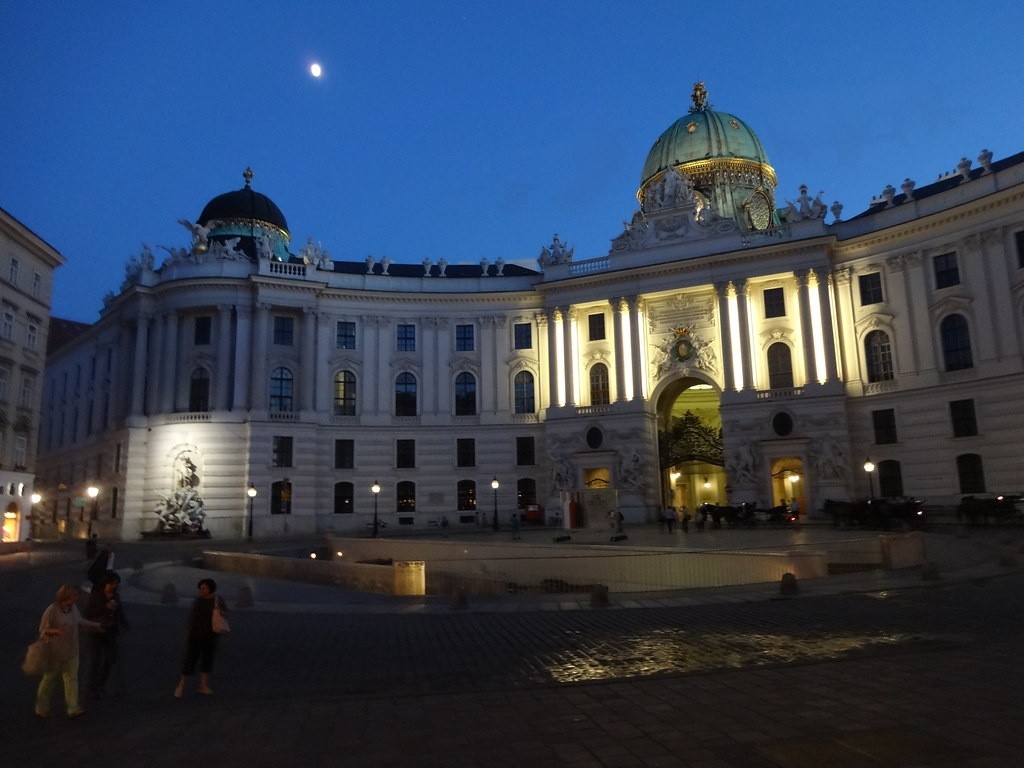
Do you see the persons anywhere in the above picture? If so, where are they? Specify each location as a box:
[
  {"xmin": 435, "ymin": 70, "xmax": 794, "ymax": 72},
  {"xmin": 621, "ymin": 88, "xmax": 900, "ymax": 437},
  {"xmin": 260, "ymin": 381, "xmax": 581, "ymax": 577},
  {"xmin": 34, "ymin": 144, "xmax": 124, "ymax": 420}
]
[
  {"xmin": 510, "ymin": 513, "xmax": 521, "ymax": 540},
  {"xmin": 476, "ymin": 510, "xmax": 492, "ymax": 530},
  {"xmin": 223, "ymin": 240, "xmax": 251, "ymax": 260},
  {"xmin": 176, "ymin": 456, "xmax": 193, "ymax": 486},
  {"xmin": 83, "ymin": 572, "xmax": 129, "ymax": 700},
  {"xmin": 90, "ymin": 543, "xmax": 115, "ymax": 600},
  {"xmin": 32, "ymin": 582, "xmax": 105, "ymax": 726},
  {"xmin": 548, "ymin": 440, "xmax": 571, "ymax": 491},
  {"xmin": 259, "ymin": 229, "xmax": 271, "ymax": 252},
  {"xmin": 694, "ymin": 505, "xmax": 706, "ymax": 532},
  {"xmin": 126, "ymin": 242, "xmax": 186, "ymax": 278},
  {"xmin": 436, "ymin": 513, "xmax": 451, "ymax": 530},
  {"xmin": 781, "ymin": 496, "xmax": 801, "ymax": 529},
  {"xmin": 85, "ymin": 534, "xmax": 98, "ymax": 559},
  {"xmin": 173, "ymin": 579, "xmax": 230, "ymax": 700},
  {"xmin": 668, "ymin": 505, "xmax": 691, "ymax": 534},
  {"xmin": 663, "ymin": 163, "xmax": 681, "ymax": 200}
]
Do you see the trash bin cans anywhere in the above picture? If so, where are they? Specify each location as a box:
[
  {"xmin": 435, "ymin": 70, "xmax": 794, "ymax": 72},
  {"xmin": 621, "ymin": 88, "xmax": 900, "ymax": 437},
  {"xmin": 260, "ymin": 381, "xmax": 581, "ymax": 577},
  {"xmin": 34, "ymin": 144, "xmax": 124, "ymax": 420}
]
[{"xmin": 392, "ymin": 560, "xmax": 426, "ymax": 597}]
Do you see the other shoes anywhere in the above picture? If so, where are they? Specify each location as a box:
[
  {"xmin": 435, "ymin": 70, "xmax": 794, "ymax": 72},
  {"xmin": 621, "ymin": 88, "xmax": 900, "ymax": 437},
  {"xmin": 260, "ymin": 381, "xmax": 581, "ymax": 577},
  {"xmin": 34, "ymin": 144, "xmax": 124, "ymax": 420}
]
[
  {"xmin": 175, "ymin": 686, "xmax": 185, "ymax": 699},
  {"xmin": 36, "ymin": 710, "xmax": 54, "ymax": 718},
  {"xmin": 198, "ymin": 686, "xmax": 213, "ymax": 695},
  {"xmin": 69, "ymin": 707, "xmax": 86, "ymax": 720}
]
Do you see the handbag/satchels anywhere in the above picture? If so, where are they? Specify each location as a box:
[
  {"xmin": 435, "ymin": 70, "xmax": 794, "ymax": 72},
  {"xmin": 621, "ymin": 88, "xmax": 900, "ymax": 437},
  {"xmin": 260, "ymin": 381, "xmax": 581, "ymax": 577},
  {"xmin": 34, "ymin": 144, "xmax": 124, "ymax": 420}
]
[
  {"xmin": 22, "ymin": 635, "xmax": 51, "ymax": 674},
  {"xmin": 212, "ymin": 596, "xmax": 230, "ymax": 635}
]
